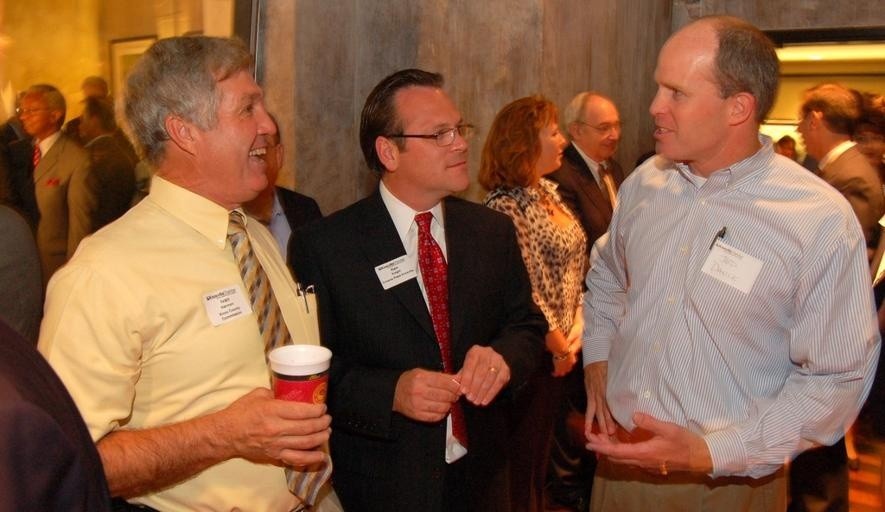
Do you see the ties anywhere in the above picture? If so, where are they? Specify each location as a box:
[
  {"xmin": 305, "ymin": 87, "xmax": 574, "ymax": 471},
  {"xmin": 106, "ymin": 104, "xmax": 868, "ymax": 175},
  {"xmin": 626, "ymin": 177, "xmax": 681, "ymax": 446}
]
[
  {"xmin": 595, "ymin": 164, "xmax": 617, "ymax": 210},
  {"xmin": 414, "ymin": 212, "xmax": 480, "ymax": 450},
  {"xmin": 29, "ymin": 140, "xmax": 43, "ymax": 169},
  {"xmin": 226, "ymin": 209, "xmax": 333, "ymax": 507}
]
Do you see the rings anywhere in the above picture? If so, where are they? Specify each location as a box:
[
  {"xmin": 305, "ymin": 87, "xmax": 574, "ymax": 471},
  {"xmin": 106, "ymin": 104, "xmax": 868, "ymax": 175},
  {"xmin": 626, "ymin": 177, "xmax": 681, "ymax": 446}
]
[{"xmin": 488, "ymin": 367, "xmax": 502, "ymax": 375}]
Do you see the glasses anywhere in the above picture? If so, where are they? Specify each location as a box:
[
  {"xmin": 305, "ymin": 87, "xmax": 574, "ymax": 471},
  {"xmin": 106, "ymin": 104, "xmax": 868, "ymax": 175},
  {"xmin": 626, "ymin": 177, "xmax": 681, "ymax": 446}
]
[
  {"xmin": 15, "ymin": 106, "xmax": 49, "ymax": 115},
  {"xmin": 579, "ymin": 118, "xmax": 622, "ymax": 134},
  {"xmin": 385, "ymin": 123, "xmax": 476, "ymax": 147}
]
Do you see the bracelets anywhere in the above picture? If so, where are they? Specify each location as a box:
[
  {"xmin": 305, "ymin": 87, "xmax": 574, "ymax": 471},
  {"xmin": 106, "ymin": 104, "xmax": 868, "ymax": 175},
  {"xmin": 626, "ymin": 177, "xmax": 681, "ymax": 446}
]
[{"xmin": 553, "ymin": 350, "xmax": 573, "ymax": 364}]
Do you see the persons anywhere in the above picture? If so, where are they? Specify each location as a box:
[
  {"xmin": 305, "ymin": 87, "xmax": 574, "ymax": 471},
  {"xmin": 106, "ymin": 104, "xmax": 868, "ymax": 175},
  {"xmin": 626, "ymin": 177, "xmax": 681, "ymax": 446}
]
[
  {"xmin": 285, "ymin": 69, "xmax": 550, "ymax": 512},
  {"xmin": 19, "ymin": 84, "xmax": 101, "ymax": 287},
  {"xmin": 795, "ymin": 83, "xmax": 885, "ymax": 284},
  {"xmin": 852, "ymin": 108, "xmax": 885, "ymax": 185},
  {"xmin": 242, "ymin": 109, "xmax": 322, "ymax": 270},
  {"xmin": 78, "ymin": 95, "xmax": 138, "ymax": 234},
  {"xmin": 0, "ymin": 94, "xmax": 42, "ymax": 231},
  {"xmin": 583, "ymin": 17, "xmax": 881, "ymax": 511},
  {"xmin": 545, "ymin": 92, "xmax": 626, "ymax": 254},
  {"xmin": 65, "ymin": 77, "xmax": 107, "ymax": 140},
  {"xmin": 774, "ymin": 135, "xmax": 795, "ymax": 160},
  {"xmin": 478, "ymin": 96, "xmax": 587, "ymax": 511},
  {"xmin": 36, "ymin": 35, "xmax": 345, "ymax": 512}
]
